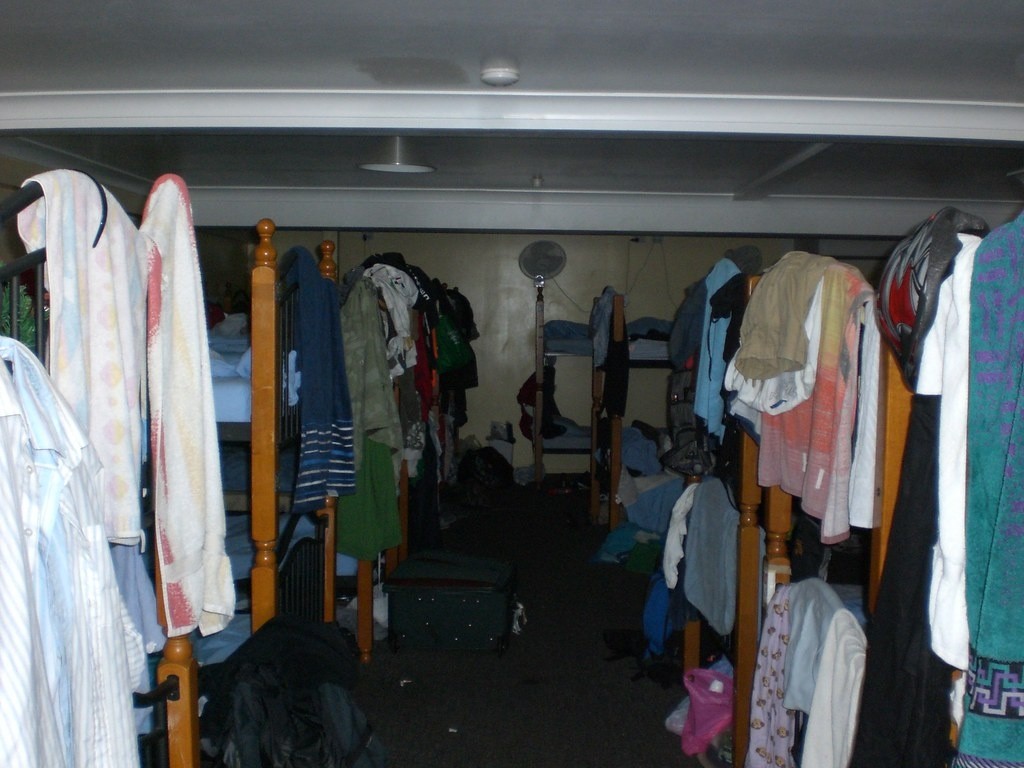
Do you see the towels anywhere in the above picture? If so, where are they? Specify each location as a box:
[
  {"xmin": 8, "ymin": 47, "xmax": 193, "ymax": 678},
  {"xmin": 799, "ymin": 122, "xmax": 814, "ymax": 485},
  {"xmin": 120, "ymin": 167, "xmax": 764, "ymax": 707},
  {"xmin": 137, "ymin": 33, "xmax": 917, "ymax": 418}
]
[
  {"xmin": 137, "ymin": 172, "xmax": 237, "ymax": 638},
  {"xmin": 268, "ymin": 252, "xmax": 413, "ymax": 562},
  {"xmin": 588, "ymin": 250, "xmax": 882, "ymax": 768},
  {"xmin": 17, "ymin": 168, "xmax": 151, "ymax": 547},
  {"xmin": 915, "ymin": 233, "xmax": 984, "ymax": 673},
  {"xmin": 951, "ymin": 211, "xmax": 1024, "ymax": 768}
]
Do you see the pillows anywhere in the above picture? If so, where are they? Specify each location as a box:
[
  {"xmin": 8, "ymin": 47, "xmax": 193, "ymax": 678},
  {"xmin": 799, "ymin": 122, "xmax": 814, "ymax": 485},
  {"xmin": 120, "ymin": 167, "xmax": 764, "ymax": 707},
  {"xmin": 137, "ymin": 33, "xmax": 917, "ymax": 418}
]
[{"xmin": 552, "ymin": 416, "xmax": 588, "ymax": 437}]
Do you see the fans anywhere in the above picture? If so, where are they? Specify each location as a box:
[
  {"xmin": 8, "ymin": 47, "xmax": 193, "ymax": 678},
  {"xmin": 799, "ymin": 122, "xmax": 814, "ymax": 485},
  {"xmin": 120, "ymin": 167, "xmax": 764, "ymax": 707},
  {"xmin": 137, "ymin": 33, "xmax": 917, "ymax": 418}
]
[{"xmin": 519, "ymin": 241, "xmax": 566, "ymax": 288}]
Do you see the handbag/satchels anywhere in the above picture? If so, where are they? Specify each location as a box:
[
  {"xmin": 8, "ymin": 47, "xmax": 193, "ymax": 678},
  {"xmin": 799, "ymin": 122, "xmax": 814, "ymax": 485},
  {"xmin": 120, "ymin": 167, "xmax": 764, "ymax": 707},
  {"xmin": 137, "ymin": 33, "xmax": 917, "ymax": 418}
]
[
  {"xmin": 681, "ymin": 669, "xmax": 735, "ymax": 757},
  {"xmin": 436, "ymin": 313, "xmax": 476, "ymax": 388}
]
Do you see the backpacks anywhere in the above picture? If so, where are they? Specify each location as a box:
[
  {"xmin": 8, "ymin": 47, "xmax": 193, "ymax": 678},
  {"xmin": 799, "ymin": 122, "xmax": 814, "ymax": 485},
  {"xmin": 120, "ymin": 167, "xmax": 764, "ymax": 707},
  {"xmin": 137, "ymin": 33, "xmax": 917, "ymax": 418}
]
[{"xmin": 212, "ymin": 659, "xmax": 385, "ymax": 768}]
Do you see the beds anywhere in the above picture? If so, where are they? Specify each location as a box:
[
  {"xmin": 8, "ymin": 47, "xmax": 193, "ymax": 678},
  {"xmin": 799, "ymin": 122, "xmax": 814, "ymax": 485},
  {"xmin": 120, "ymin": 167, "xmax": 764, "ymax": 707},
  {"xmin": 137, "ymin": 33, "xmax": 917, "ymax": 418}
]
[
  {"xmin": 0, "ymin": 171, "xmax": 459, "ymax": 768},
  {"xmin": 535, "ymin": 207, "xmax": 1024, "ymax": 768}
]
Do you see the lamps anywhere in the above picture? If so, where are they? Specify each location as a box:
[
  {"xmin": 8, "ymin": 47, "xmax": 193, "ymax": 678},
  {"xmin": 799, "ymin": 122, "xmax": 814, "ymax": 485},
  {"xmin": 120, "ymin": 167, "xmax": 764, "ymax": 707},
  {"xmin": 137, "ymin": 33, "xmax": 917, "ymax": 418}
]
[{"xmin": 357, "ymin": 137, "xmax": 437, "ymax": 173}]
